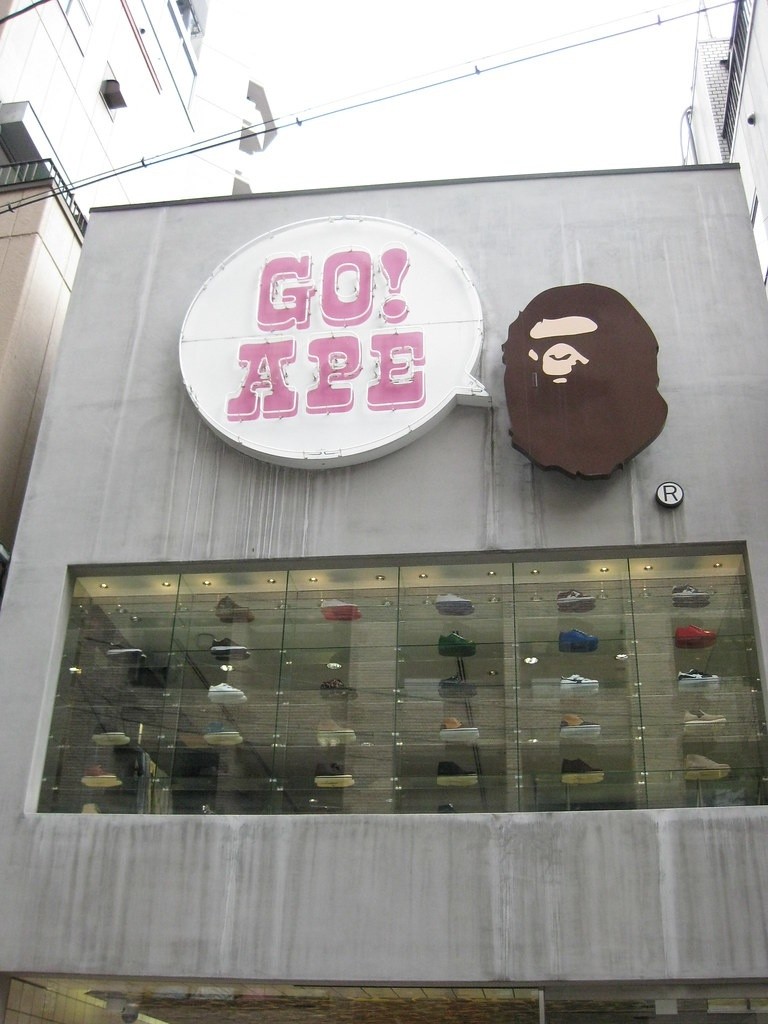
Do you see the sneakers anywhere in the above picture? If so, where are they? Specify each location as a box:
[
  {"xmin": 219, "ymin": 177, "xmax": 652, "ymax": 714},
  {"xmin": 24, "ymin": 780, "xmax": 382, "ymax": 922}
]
[
  {"xmin": 208, "ymin": 722, "xmax": 240, "ymax": 735},
  {"xmin": 106, "ymin": 644, "xmax": 146, "ymax": 661},
  {"xmin": 82, "ymin": 764, "xmax": 116, "ymax": 778},
  {"xmin": 557, "ymin": 589, "xmax": 596, "ymax": 613},
  {"xmin": 673, "ymin": 583, "xmax": 731, "ymax": 781},
  {"xmin": 561, "ymin": 714, "xmax": 601, "ymax": 736},
  {"xmin": 320, "ymin": 678, "xmax": 357, "ymax": 700},
  {"xmin": 560, "ymin": 673, "xmax": 598, "ymax": 693},
  {"xmin": 219, "ymin": 597, "xmax": 255, "ymax": 623},
  {"xmin": 321, "ymin": 598, "xmax": 361, "ymax": 620},
  {"xmin": 562, "ymin": 758, "xmax": 605, "ymax": 784},
  {"xmin": 316, "ymin": 760, "xmax": 352, "ymax": 778},
  {"xmin": 209, "ymin": 682, "xmax": 247, "ymax": 704},
  {"xmin": 95, "ymin": 723, "xmax": 125, "ymax": 736},
  {"xmin": 559, "ymin": 628, "xmax": 598, "ymax": 653},
  {"xmin": 318, "ymin": 718, "xmax": 355, "ymax": 734},
  {"xmin": 211, "ymin": 638, "xmax": 250, "ymax": 660}
]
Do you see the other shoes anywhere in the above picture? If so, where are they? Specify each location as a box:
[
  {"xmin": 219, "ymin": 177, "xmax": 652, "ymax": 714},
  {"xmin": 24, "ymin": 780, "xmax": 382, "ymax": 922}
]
[{"xmin": 434, "ymin": 591, "xmax": 479, "ymax": 814}]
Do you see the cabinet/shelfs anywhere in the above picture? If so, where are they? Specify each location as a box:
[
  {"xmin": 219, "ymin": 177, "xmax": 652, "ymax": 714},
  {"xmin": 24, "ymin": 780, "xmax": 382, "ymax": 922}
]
[{"xmin": 38, "ymin": 590, "xmax": 767, "ymax": 815}]
